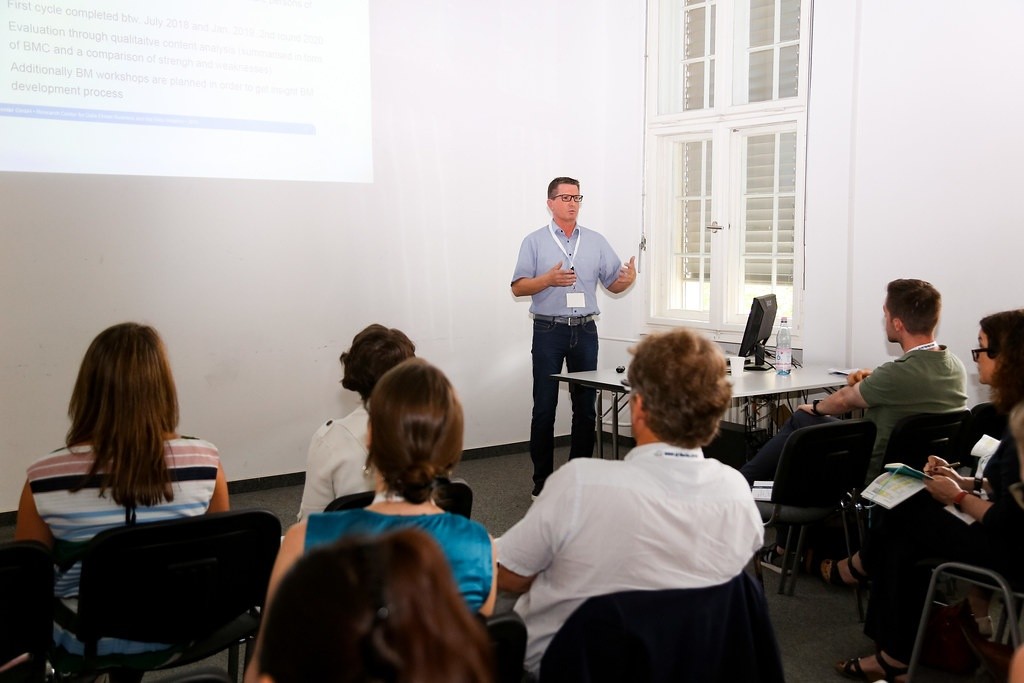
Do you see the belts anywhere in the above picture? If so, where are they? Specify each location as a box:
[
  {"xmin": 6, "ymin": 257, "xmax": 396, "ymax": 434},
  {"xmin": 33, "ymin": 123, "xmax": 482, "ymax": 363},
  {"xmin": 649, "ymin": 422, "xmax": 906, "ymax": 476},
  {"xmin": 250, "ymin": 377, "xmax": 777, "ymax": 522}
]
[{"xmin": 533, "ymin": 313, "xmax": 594, "ymax": 326}]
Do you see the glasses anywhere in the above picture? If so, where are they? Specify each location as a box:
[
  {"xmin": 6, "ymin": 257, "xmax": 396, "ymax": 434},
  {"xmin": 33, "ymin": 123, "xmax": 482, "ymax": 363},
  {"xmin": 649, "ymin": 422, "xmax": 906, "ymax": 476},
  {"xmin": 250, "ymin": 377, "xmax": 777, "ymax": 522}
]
[
  {"xmin": 972, "ymin": 347, "xmax": 999, "ymax": 362},
  {"xmin": 551, "ymin": 194, "xmax": 583, "ymax": 202}
]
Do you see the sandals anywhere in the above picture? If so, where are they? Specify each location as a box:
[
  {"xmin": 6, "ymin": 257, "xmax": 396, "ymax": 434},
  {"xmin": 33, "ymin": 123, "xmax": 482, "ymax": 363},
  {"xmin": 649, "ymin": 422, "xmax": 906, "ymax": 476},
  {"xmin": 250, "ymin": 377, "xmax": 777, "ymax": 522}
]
[
  {"xmin": 818, "ymin": 553, "xmax": 870, "ymax": 590},
  {"xmin": 833, "ymin": 651, "xmax": 909, "ymax": 683}
]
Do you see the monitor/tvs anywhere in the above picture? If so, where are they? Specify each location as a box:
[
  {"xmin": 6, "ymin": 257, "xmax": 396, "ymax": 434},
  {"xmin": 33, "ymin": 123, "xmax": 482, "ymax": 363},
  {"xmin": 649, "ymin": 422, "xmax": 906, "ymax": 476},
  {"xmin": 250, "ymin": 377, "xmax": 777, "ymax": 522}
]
[{"xmin": 738, "ymin": 293, "xmax": 778, "ymax": 371}]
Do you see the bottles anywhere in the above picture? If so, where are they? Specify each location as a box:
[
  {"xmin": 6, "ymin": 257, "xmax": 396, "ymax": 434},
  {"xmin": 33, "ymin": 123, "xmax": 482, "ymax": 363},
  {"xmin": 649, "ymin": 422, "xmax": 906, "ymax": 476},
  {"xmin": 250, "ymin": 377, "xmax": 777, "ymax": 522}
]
[{"xmin": 775, "ymin": 317, "xmax": 792, "ymax": 375}]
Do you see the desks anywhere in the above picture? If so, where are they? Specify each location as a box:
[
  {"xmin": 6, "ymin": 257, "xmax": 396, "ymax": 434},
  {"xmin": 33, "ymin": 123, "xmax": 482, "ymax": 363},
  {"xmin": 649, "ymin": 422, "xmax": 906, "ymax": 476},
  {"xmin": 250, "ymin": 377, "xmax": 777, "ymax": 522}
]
[{"xmin": 544, "ymin": 355, "xmax": 862, "ymax": 463}]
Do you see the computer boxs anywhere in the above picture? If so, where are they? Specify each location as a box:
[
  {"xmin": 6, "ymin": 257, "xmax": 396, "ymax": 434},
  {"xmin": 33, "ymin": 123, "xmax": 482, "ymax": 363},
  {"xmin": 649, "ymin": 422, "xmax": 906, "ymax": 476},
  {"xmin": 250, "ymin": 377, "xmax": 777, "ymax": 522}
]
[{"xmin": 699, "ymin": 421, "xmax": 768, "ymax": 470}]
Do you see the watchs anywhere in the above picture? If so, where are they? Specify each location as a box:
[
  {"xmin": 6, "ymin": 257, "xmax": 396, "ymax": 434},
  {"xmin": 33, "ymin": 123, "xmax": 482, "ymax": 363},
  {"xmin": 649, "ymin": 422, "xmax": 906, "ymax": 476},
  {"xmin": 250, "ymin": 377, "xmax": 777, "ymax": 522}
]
[
  {"xmin": 810, "ymin": 399, "xmax": 824, "ymax": 417},
  {"xmin": 952, "ymin": 490, "xmax": 969, "ymax": 513}
]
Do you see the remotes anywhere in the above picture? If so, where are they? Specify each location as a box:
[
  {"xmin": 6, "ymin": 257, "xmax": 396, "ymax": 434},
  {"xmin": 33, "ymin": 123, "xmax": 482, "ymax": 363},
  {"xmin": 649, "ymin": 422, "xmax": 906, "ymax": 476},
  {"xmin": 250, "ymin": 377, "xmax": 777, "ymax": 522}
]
[{"xmin": 615, "ymin": 365, "xmax": 625, "ymax": 373}]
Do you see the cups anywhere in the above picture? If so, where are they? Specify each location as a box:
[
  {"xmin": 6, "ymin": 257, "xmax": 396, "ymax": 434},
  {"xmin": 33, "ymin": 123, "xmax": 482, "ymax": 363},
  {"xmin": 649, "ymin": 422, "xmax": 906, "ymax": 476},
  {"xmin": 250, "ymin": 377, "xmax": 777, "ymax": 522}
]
[{"xmin": 729, "ymin": 357, "xmax": 745, "ymax": 378}]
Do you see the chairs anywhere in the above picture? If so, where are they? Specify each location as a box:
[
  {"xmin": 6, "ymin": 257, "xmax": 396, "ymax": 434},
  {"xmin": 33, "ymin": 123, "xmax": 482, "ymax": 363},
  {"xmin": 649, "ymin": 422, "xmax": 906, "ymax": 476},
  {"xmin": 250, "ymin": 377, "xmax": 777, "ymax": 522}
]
[
  {"xmin": 745, "ymin": 400, "xmax": 1024, "ymax": 682},
  {"xmin": 0, "ymin": 479, "xmax": 777, "ymax": 681}
]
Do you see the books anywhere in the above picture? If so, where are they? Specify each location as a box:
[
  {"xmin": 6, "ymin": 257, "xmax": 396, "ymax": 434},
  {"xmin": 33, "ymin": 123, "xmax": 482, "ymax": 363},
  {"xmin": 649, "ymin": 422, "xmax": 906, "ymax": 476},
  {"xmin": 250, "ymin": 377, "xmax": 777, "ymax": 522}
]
[{"xmin": 884, "ymin": 462, "xmax": 934, "ymax": 483}]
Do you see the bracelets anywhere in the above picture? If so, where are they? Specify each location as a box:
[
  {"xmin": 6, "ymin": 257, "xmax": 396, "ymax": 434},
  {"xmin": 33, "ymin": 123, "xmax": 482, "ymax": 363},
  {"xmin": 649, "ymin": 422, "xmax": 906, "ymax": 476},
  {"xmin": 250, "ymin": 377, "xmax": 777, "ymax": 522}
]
[{"xmin": 972, "ymin": 476, "xmax": 983, "ymax": 498}]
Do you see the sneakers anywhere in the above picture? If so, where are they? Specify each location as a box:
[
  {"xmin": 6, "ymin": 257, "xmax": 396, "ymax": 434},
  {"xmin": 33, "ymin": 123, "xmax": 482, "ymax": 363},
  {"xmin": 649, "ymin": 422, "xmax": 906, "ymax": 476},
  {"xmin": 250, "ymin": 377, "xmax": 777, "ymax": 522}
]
[{"xmin": 758, "ymin": 543, "xmax": 803, "ymax": 575}]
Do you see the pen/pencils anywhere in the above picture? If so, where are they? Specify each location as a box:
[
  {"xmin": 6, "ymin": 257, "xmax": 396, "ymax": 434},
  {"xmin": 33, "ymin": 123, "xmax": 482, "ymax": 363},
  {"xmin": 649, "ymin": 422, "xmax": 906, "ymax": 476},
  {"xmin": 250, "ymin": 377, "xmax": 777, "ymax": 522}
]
[{"xmin": 925, "ymin": 462, "xmax": 960, "ymax": 472}]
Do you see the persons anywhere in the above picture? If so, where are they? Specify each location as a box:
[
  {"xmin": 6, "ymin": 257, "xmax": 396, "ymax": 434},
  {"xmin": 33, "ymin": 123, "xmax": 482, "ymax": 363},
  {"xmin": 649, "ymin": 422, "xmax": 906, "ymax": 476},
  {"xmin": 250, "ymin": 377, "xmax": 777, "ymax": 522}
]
[
  {"xmin": 12, "ymin": 321, "xmax": 234, "ymax": 683},
  {"xmin": 740, "ymin": 278, "xmax": 967, "ymax": 577},
  {"xmin": 499, "ymin": 329, "xmax": 766, "ymax": 683},
  {"xmin": 509, "ymin": 176, "xmax": 637, "ymax": 501},
  {"xmin": 292, "ymin": 324, "xmax": 417, "ymax": 520},
  {"xmin": 242, "ymin": 356, "xmax": 497, "ymax": 683},
  {"xmin": 819, "ymin": 308, "xmax": 1024, "ymax": 683}
]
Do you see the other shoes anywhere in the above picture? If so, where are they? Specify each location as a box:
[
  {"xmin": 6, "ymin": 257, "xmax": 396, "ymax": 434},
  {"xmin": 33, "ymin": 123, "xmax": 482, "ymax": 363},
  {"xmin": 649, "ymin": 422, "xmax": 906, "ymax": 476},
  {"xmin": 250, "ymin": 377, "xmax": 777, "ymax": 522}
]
[
  {"xmin": 531, "ymin": 484, "xmax": 543, "ymax": 501},
  {"xmin": 971, "ymin": 615, "xmax": 993, "ymax": 639}
]
